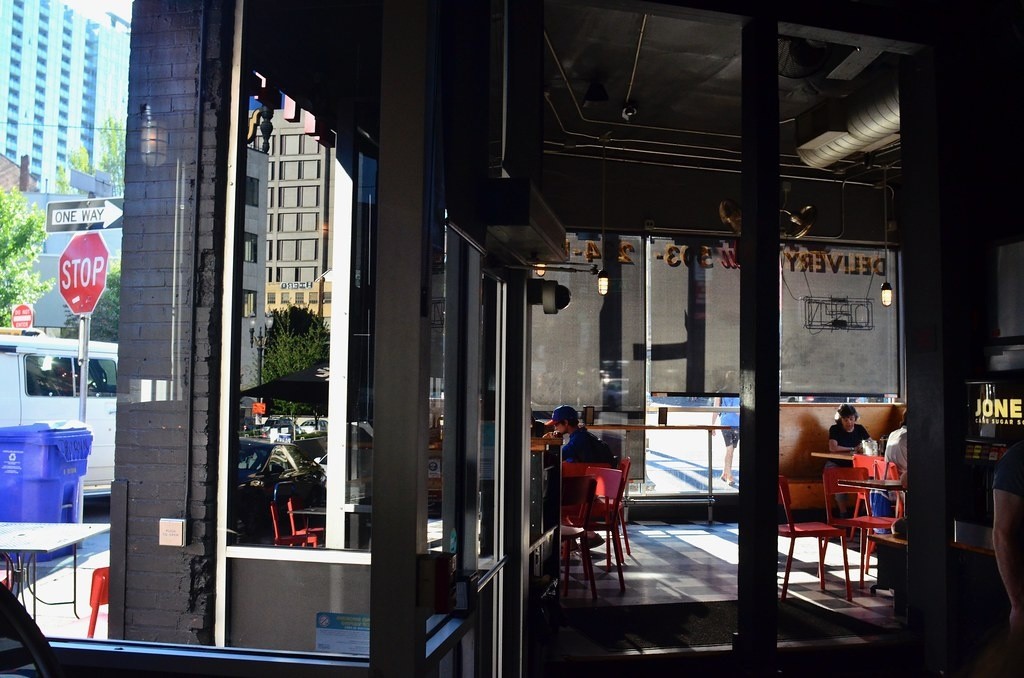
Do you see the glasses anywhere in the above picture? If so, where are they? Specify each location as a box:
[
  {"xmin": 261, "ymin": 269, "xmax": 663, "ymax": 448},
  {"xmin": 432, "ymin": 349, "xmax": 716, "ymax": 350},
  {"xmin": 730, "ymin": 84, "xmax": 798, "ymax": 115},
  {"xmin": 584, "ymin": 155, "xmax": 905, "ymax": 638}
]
[{"xmin": 554, "ymin": 420, "xmax": 566, "ymax": 427}]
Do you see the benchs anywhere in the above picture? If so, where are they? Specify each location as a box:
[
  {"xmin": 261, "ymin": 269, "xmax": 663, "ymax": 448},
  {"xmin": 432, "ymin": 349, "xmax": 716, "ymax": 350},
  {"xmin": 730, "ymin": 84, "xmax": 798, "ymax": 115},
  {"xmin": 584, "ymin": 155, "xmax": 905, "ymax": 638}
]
[{"xmin": 779, "ymin": 479, "xmax": 866, "ymax": 510}]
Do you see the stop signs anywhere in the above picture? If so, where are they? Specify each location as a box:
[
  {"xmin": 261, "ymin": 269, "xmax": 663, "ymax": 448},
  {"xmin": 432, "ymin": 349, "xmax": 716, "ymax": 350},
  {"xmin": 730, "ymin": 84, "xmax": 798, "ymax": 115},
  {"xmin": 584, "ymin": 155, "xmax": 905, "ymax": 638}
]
[
  {"xmin": 58, "ymin": 232, "xmax": 109, "ymax": 315},
  {"xmin": 11, "ymin": 304, "xmax": 33, "ymax": 328}
]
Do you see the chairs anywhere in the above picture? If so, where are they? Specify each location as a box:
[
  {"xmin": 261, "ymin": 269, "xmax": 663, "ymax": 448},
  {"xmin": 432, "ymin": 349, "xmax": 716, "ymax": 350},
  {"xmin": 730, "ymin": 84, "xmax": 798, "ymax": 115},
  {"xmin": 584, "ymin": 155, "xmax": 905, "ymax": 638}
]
[
  {"xmin": 269, "ymin": 481, "xmax": 325, "ymax": 548},
  {"xmin": 778, "ymin": 453, "xmax": 904, "ymax": 602},
  {"xmin": 561, "ymin": 457, "xmax": 631, "ymax": 600}
]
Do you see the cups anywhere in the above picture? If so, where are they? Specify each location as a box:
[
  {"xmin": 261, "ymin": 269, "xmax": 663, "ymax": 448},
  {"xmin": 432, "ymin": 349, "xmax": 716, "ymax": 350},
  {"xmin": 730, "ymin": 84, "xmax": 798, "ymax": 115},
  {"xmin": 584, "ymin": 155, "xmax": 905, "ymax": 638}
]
[{"xmin": 862, "ymin": 440, "xmax": 879, "ymax": 455}]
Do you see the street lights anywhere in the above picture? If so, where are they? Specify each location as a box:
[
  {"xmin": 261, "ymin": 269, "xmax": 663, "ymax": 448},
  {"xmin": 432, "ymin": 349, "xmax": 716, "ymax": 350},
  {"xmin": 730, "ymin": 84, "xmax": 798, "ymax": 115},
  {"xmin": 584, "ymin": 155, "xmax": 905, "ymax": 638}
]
[{"xmin": 249, "ymin": 318, "xmax": 274, "ymax": 403}]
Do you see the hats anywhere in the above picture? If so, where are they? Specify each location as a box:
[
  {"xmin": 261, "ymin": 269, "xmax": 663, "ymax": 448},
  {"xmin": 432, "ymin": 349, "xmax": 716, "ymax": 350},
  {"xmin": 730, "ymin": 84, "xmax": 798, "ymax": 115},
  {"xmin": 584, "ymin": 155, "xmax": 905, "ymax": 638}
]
[{"xmin": 552, "ymin": 405, "xmax": 578, "ymax": 420}]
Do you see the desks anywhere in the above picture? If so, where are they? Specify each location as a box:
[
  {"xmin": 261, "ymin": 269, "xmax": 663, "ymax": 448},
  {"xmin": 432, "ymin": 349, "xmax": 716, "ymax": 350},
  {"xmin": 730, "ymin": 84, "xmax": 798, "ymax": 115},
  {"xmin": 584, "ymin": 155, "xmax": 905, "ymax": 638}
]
[
  {"xmin": 810, "ymin": 452, "xmax": 854, "ymax": 460},
  {"xmin": 0, "ymin": 522, "xmax": 111, "ymax": 623},
  {"xmin": 838, "ymin": 479, "xmax": 904, "ymax": 491},
  {"xmin": 866, "ymin": 533, "xmax": 907, "ymax": 596}
]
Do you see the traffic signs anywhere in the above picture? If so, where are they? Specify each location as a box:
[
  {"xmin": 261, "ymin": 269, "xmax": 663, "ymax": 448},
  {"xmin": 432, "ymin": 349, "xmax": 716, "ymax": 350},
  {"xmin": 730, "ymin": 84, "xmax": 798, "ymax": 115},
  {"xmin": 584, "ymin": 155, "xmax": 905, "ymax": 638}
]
[{"xmin": 45, "ymin": 196, "xmax": 123, "ymax": 231}]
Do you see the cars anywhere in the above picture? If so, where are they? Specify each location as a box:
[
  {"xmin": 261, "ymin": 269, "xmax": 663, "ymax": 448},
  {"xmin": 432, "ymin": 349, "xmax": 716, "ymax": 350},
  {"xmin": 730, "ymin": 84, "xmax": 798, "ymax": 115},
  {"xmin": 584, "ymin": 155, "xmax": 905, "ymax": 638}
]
[
  {"xmin": 300, "ymin": 420, "xmax": 328, "ymax": 434},
  {"xmin": 261, "ymin": 418, "xmax": 303, "ymax": 441},
  {"xmin": 233, "ymin": 438, "xmax": 326, "ymax": 537},
  {"xmin": 243, "ymin": 417, "xmax": 255, "ymax": 431},
  {"xmin": 314, "ymin": 449, "xmax": 373, "ymax": 504}
]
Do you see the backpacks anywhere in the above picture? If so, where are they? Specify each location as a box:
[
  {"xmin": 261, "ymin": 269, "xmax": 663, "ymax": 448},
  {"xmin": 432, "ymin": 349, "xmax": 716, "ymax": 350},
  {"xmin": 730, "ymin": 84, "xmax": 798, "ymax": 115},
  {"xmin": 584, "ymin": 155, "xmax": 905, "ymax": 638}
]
[{"xmin": 869, "ymin": 462, "xmax": 891, "ymax": 534}]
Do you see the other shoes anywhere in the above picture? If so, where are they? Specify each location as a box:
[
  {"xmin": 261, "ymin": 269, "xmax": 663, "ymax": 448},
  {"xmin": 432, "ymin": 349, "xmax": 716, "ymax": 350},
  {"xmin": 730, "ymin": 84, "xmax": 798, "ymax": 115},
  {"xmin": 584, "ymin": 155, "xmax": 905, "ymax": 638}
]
[
  {"xmin": 839, "ymin": 513, "xmax": 860, "ymax": 537},
  {"xmin": 720, "ymin": 472, "xmax": 735, "ymax": 486}
]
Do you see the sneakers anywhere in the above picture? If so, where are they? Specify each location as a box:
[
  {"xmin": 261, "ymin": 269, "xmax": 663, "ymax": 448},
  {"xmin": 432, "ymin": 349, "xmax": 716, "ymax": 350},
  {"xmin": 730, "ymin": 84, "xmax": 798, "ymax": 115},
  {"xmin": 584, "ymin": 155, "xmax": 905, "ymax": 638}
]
[
  {"xmin": 561, "ymin": 541, "xmax": 578, "ymax": 551},
  {"xmin": 579, "ymin": 533, "xmax": 605, "ymax": 550}
]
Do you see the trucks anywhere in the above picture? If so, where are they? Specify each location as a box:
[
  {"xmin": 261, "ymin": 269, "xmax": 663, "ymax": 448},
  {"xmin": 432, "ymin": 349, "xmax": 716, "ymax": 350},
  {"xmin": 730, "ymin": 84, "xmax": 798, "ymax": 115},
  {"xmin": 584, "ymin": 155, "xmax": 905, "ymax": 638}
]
[{"xmin": 0, "ymin": 335, "xmax": 118, "ymax": 499}]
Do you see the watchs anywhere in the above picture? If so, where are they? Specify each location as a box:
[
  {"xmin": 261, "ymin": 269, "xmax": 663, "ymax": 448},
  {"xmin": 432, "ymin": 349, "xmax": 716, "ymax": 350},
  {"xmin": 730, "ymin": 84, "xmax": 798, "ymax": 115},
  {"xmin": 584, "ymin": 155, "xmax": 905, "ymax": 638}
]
[{"xmin": 851, "ymin": 447, "xmax": 855, "ymax": 452}]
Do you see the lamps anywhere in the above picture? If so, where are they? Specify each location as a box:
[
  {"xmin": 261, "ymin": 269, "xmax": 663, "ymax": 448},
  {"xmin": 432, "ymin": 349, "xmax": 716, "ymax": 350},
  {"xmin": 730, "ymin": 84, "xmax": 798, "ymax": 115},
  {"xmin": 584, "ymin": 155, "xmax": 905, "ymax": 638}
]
[
  {"xmin": 597, "ymin": 136, "xmax": 609, "ymax": 294},
  {"xmin": 881, "ymin": 170, "xmax": 893, "ymax": 306}
]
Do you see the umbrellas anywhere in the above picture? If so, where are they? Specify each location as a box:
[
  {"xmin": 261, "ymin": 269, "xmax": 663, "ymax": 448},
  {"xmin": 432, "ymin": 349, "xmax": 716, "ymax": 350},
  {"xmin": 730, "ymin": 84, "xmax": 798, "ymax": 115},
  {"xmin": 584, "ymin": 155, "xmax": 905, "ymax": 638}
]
[{"xmin": 241, "ymin": 355, "xmax": 329, "ymax": 430}]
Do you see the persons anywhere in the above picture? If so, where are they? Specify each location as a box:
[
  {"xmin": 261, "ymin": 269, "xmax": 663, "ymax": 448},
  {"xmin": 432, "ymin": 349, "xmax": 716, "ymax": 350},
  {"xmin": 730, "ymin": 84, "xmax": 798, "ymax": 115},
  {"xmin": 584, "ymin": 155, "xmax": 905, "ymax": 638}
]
[
  {"xmin": 884, "ymin": 407, "xmax": 907, "ymax": 473},
  {"xmin": 825, "ymin": 404, "xmax": 873, "ymax": 529},
  {"xmin": 712, "ymin": 371, "xmax": 740, "ymax": 486},
  {"xmin": 530, "ymin": 406, "xmax": 618, "ymax": 552},
  {"xmin": 990, "ymin": 439, "xmax": 1024, "ymax": 651}
]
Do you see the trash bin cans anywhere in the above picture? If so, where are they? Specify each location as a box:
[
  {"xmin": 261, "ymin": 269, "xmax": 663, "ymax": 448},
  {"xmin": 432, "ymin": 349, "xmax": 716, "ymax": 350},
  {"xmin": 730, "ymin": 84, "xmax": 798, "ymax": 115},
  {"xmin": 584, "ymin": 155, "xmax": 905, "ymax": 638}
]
[{"xmin": 0, "ymin": 420, "xmax": 93, "ymax": 563}]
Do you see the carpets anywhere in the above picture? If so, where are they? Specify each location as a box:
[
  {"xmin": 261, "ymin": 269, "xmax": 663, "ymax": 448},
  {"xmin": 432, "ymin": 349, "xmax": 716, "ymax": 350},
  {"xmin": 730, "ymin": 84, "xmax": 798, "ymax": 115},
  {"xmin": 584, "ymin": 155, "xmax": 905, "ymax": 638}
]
[{"xmin": 563, "ymin": 596, "xmax": 900, "ymax": 653}]
